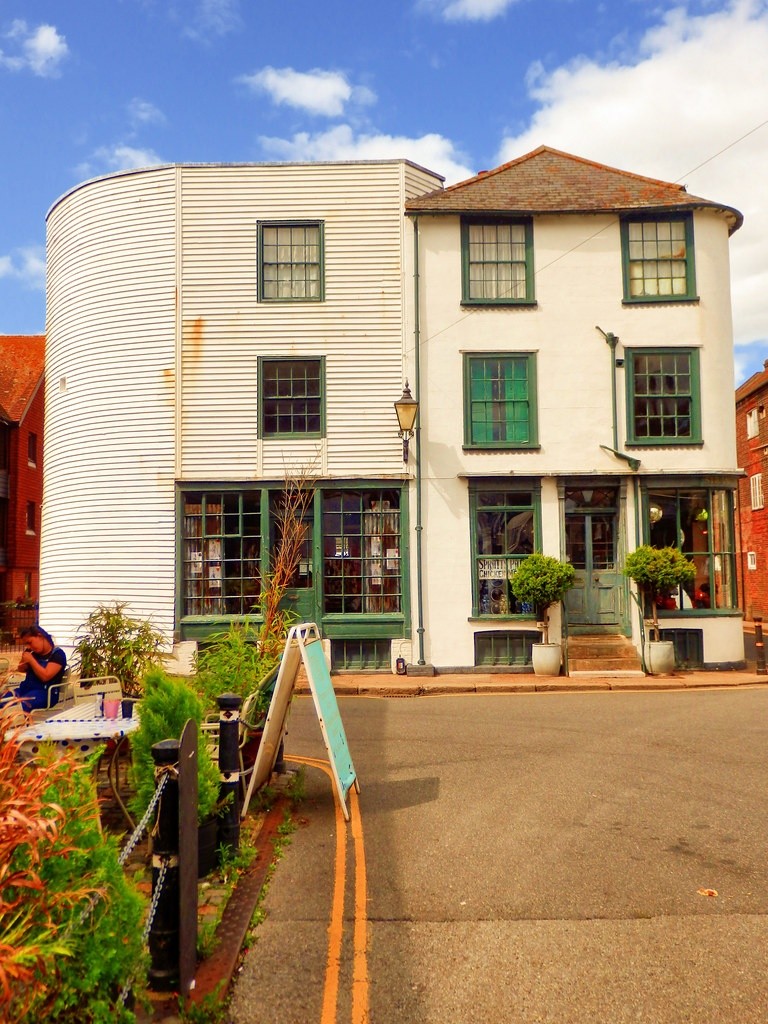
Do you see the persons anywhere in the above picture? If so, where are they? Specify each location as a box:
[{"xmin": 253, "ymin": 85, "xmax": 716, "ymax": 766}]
[{"xmin": 0, "ymin": 624, "xmax": 66, "ymax": 721}]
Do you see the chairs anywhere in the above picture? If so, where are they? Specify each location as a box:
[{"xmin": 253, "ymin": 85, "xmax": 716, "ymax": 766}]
[
  {"xmin": 201, "ymin": 690, "xmax": 259, "ymax": 799},
  {"xmin": 0, "ymin": 657, "xmax": 123, "ymax": 724}
]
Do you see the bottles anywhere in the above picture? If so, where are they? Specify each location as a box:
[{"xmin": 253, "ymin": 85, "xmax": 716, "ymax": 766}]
[
  {"xmin": 94, "ymin": 692, "xmax": 104, "ymax": 718},
  {"xmin": 396, "ymin": 654, "xmax": 405, "ymax": 675}
]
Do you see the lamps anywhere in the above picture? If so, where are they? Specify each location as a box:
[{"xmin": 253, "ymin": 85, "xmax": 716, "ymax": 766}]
[{"xmin": 395, "ymin": 381, "xmax": 419, "ymax": 462}]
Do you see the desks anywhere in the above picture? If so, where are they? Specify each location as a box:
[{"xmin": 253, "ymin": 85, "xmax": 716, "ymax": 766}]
[{"xmin": 5, "ymin": 702, "xmax": 142, "ymax": 844}]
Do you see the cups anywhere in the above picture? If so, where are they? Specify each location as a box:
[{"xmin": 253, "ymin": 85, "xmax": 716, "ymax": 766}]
[{"xmin": 104, "ymin": 697, "xmax": 120, "ymax": 719}]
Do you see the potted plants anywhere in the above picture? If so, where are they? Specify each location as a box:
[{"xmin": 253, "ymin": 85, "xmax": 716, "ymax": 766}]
[
  {"xmin": 622, "ymin": 543, "xmax": 697, "ymax": 675},
  {"xmin": 126, "ymin": 568, "xmax": 303, "ymax": 877},
  {"xmin": 509, "ymin": 553, "xmax": 576, "ymax": 676}
]
